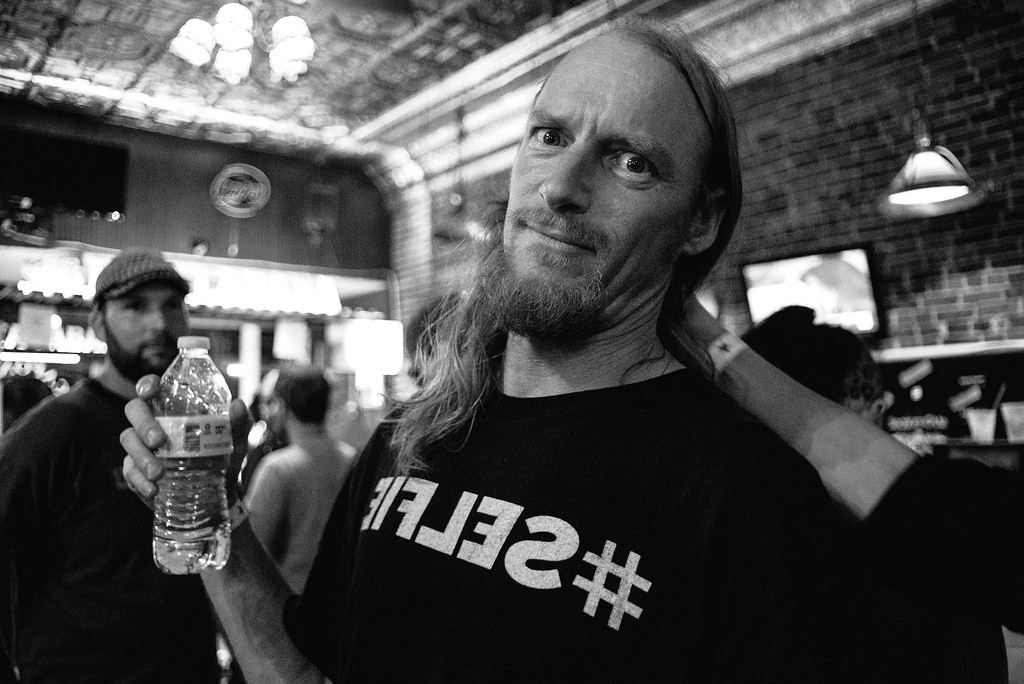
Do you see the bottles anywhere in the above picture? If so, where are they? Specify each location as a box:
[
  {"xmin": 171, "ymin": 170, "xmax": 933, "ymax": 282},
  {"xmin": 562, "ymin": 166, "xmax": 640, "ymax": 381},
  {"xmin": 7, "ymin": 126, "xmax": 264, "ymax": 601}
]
[{"xmin": 152, "ymin": 337, "xmax": 233, "ymax": 575}]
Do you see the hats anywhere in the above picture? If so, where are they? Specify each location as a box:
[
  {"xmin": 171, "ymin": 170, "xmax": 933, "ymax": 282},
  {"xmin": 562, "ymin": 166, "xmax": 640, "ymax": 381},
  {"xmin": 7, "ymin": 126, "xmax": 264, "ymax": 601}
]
[{"xmin": 93, "ymin": 250, "xmax": 191, "ymax": 307}]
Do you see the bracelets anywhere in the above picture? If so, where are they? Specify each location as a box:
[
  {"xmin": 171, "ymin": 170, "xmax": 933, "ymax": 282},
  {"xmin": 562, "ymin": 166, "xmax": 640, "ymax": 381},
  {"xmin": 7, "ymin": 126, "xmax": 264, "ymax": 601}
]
[
  {"xmin": 704, "ymin": 333, "xmax": 748, "ymax": 382},
  {"xmin": 229, "ymin": 500, "xmax": 248, "ymax": 530}
]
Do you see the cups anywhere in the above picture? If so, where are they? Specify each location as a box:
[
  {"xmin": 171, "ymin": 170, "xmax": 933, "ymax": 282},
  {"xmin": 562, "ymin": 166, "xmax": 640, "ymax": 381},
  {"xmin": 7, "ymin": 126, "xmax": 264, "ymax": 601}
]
[
  {"xmin": 1001, "ymin": 402, "xmax": 1024, "ymax": 443},
  {"xmin": 968, "ymin": 408, "xmax": 996, "ymax": 442}
]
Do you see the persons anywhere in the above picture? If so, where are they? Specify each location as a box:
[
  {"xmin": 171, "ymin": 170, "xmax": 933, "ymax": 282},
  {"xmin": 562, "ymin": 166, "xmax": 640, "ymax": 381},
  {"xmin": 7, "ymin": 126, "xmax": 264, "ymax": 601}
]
[
  {"xmin": 667, "ymin": 292, "xmax": 1024, "ymax": 639},
  {"xmin": 0, "ymin": 248, "xmax": 223, "ymax": 684},
  {"xmin": 119, "ymin": 16, "xmax": 1008, "ymax": 684},
  {"xmin": 242, "ymin": 365, "xmax": 357, "ymax": 596}
]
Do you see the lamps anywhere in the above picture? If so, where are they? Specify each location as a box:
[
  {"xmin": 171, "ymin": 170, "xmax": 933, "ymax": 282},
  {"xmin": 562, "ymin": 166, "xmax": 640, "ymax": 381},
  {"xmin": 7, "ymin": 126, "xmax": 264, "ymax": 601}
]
[
  {"xmin": 170, "ymin": 0, "xmax": 316, "ymax": 86},
  {"xmin": 888, "ymin": 0, "xmax": 971, "ymax": 210}
]
